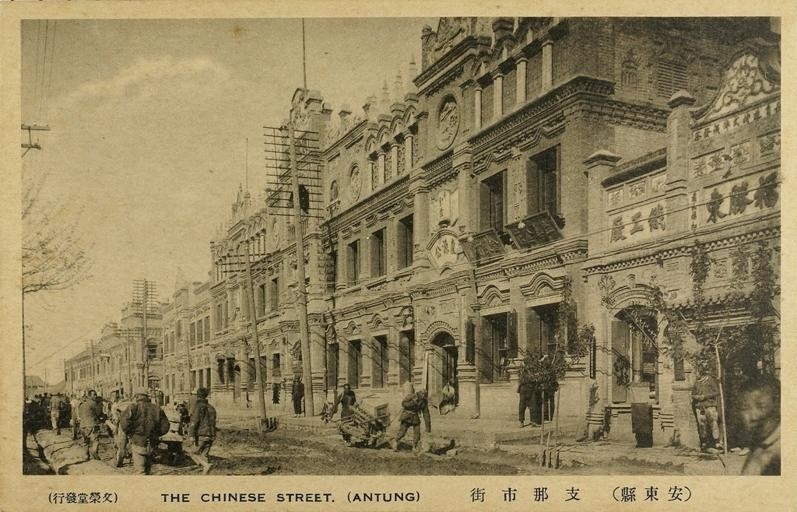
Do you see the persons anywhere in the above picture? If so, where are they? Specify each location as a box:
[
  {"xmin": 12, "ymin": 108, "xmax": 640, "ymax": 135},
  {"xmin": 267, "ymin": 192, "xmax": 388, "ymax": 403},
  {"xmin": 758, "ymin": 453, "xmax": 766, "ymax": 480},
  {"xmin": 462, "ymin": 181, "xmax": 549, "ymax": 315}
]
[
  {"xmin": 516, "ymin": 355, "xmax": 558, "ymax": 428},
  {"xmin": 691, "ymin": 361, "xmax": 724, "ymax": 453},
  {"xmin": 120, "ymin": 387, "xmax": 170, "ymax": 475},
  {"xmin": 333, "ymin": 384, "xmax": 356, "ymax": 445},
  {"xmin": 391, "ymin": 389, "xmax": 431, "ymax": 452},
  {"xmin": 23, "ymin": 390, "xmax": 127, "ymax": 467},
  {"xmin": 291, "ymin": 376, "xmax": 304, "ymax": 418},
  {"xmin": 737, "ymin": 376, "xmax": 781, "ymax": 476},
  {"xmin": 181, "ymin": 389, "xmax": 216, "ymax": 475}
]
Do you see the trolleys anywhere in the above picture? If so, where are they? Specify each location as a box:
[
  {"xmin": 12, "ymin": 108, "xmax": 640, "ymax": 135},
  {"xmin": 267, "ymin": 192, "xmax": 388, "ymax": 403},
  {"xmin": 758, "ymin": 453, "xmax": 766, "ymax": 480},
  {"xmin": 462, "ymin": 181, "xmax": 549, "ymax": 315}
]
[{"xmin": 330, "ymin": 391, "xmax": 391, "ymax": 446}]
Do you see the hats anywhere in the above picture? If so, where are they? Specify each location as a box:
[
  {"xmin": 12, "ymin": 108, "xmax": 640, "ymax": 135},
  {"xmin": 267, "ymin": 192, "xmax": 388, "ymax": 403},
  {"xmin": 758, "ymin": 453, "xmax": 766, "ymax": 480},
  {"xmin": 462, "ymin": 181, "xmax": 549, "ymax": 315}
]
[{"xmin": 134, "ymin": 386, "xmax": 151, "ymax": 397}]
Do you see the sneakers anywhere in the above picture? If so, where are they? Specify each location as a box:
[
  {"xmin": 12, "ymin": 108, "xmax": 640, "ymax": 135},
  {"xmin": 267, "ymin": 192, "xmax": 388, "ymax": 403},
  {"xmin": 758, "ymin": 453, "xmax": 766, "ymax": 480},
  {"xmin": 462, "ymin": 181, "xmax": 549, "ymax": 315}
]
[
  {"xmin": 715, "ymin": 440, "xmax": 723, "ymax": 450},
  {"xmin": 518, "ymin": 420, "xmax": 552, "ymax": 428},
  {"xmin": 391, "ymin": 439, "xmax": 399, "ymax": 451},
  {"xmin": 202, "ymin": 463, "xmax": 214, "ymax": 475},
  {"xmin": 116, "ymin": 455, "xmax": 123, "ymax": 467},
  {"xmin": 83, "ymin": 451, "xmax": 101, "ymax": 461}
]
